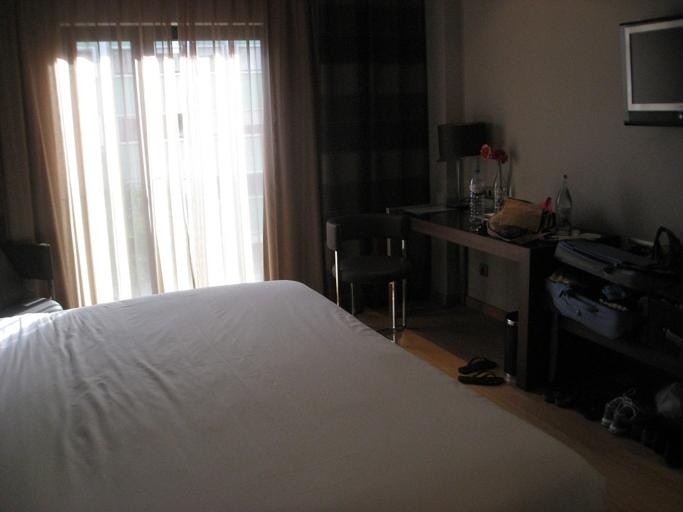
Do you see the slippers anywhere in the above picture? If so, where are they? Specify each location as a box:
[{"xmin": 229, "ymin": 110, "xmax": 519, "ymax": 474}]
[
  {"xmin": 457, "ymin": 355, "xmax": 498, "ymax": 375},
  {"xmin": 458, "ymin": 371, "xmax": 505, "ymax": 386}
]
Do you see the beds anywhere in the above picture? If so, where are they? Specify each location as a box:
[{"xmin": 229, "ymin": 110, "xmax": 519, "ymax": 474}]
[{"xmin": 0, "ymin": 277, "xmax": 612, "ymax": 512}]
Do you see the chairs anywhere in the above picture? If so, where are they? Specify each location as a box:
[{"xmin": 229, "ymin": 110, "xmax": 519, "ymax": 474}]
[
  {"xmin": 0, "ymin": 237, "xmax": 63, "ymax": 315},
  {"xmin": 325, "ymin": 209, "xmax": 412, "ymax": 330}
]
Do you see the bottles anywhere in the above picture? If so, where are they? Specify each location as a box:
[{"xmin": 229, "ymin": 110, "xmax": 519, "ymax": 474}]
[
  {"xmin": 554, "ymin": 174, "xmax": 572, "ymax": 236},
  {"xmin": 468, "ymin": 169, "xmax": 485, "ymax": 225}
]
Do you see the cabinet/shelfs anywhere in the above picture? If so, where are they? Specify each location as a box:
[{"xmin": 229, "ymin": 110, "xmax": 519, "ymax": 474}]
[{"xmin": 543, "ymin": 231, "xmax": 681, "ymax": 405}]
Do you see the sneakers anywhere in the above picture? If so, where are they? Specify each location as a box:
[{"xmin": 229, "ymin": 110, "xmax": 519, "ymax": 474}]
[{"xmin": 542, "ymin": 380, "xmax": 683, "ymax": 471}]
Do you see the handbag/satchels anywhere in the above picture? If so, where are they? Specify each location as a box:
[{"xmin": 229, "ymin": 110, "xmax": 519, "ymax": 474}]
[{"xmin": 485, "ymin": 196, "xmax": 551, "ymax": 245}]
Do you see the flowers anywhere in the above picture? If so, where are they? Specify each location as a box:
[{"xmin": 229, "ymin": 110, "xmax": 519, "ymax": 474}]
[{"xmin": 479, "ymin": 142, "xmax": 509, "ymax": 193}]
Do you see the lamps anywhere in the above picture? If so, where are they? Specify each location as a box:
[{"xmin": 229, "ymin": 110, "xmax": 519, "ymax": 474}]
[{"xmin": 435, "ymin": 120, "xmax": 493, "ymax": 210}]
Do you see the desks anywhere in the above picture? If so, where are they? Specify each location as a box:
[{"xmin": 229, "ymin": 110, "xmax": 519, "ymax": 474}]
[{"xmin": 391, "ymin": 198, "xmax": 619, "ymax": 391}]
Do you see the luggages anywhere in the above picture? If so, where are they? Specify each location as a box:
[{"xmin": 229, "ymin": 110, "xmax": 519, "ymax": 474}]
[{"xmin": 542, "ymin": 233, "xmax": 657, "ymax": 341}]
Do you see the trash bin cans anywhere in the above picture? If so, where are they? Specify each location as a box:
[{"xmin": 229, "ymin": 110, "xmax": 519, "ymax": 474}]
[{"xmin": 503, "ymin": 311, "xmax": 519, "ymax": 377}]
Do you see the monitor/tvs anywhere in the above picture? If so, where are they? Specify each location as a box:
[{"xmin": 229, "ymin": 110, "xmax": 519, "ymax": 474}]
[{"xmin": 624, "ymin": 19, "xmax": 683, "ymax": 122}]
